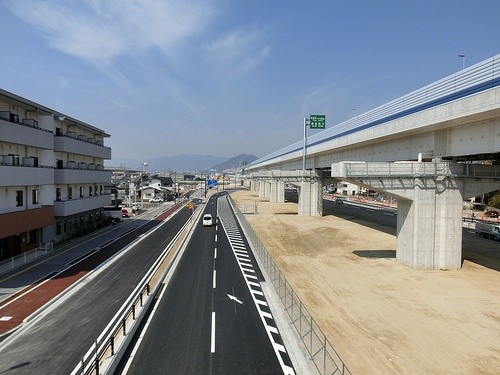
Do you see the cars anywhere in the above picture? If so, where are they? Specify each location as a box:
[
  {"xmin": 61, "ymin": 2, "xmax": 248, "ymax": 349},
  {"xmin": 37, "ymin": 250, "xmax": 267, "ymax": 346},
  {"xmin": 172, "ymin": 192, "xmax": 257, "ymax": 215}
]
[
  {"xmin": 148, "ymin": 196, "xmax": 164, "ymax": 203},
  {"xmin": 122, "ymin": 209, "xmax": 129, "ymax": 217},
  {"xmin": 485, "ymin": 210, "xmax": 499, "ymax": 218}
]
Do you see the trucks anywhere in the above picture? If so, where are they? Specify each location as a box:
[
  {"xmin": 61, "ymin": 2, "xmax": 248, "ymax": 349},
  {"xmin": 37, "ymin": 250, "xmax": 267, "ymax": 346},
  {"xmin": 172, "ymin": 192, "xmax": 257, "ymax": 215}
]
[{"xmin": 475, "ymin": 220, "xmax": 500, "ymax": 243}]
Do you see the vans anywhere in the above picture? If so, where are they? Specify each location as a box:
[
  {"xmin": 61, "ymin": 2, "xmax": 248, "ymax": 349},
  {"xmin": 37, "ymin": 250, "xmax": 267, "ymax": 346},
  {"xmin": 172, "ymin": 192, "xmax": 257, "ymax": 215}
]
[{"xmin": 202, "ymin": 213, "xmax": 214, "ymax": 227}]
